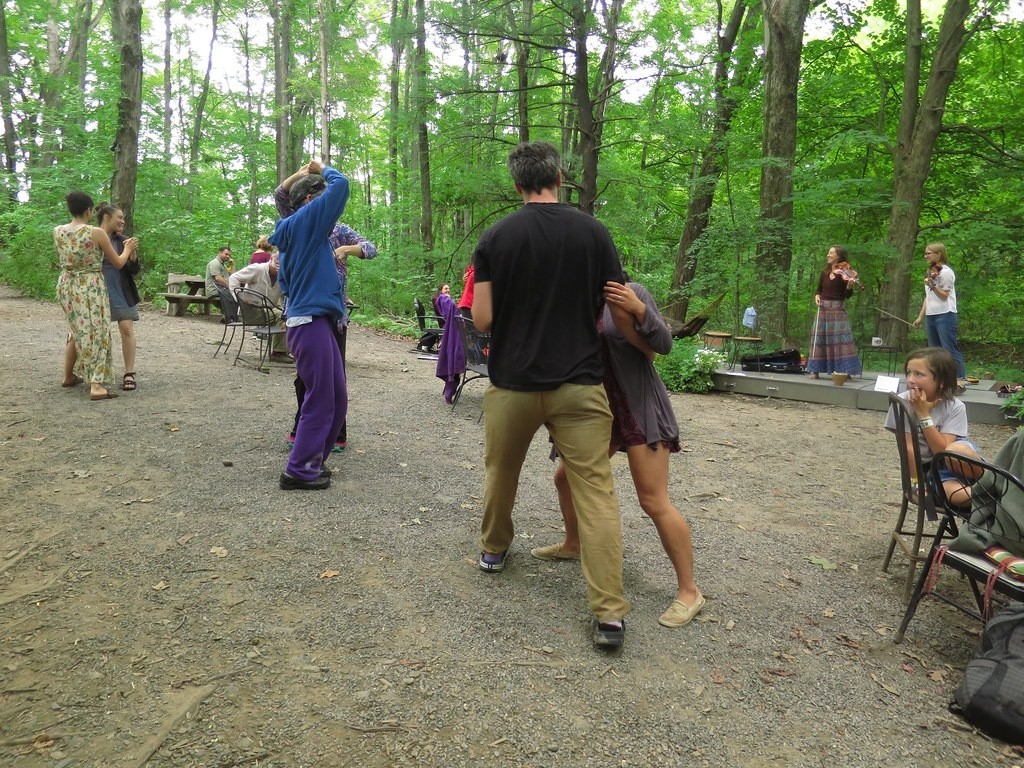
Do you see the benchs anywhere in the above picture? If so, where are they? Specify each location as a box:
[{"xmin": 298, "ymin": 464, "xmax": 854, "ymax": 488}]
[{"xmin": 154, "ymin": 293, "xmax": 220, "ymax": 304}]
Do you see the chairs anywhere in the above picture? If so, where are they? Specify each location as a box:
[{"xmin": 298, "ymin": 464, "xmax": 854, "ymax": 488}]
[
  {"xmin": 231, "ymin": 287, "xmax": 287, "ymax": 367},
  {"xmin": 881, "ymin": 389, "xmax": 986, "ymax": 604},
  {"xmin": 414, "ymin": 296, "xmax": 447, "ymax": 353},
  {"xmin": 892, "ymin": 449, "xmax": 1024, "ymax": 657},
  {"xmin": 212, "ymin": 281, "xmax": 273, "ymax": 363},
  {"xmin": 165, "ymin": 272, "xmax": 204, "ymax": 314},
  {"xmin": 450, "ymin": 315, "xmax": 491, "ymax": 423}
]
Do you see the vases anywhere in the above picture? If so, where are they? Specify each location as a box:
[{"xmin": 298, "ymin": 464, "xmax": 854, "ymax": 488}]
[{"xmin": 830, "ymin": 373, "xmax": 848, "ymax": 386}]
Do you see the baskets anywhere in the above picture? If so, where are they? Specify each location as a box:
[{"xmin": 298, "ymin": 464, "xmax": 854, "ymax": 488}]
[{"xmin": 831, "ymin": 372, "xmax": 847, "ymax": 385}]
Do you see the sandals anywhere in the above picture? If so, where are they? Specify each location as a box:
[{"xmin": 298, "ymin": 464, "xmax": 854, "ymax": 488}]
[{"xmin": 122, "ymin": 372, "xmax": 137, "ymax": 390}]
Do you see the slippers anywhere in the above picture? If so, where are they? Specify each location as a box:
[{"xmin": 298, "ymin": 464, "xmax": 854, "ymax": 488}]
[
  {"xmin": 90, "ymin": 388, "xmax": 119, "ymax": 401},
  {"xmin": 61, "ymin": 374, "xmax": 84, "ymax": 387}
]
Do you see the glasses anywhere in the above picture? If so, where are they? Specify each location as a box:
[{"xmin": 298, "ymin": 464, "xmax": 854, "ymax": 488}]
[{"xmin": 925, "ymin": 252, "xmax": 938, "ymax": 256}]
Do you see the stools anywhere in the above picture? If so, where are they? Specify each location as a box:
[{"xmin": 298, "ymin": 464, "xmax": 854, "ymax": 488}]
[
  {"xmin": 728, "ymin": 336, "xmax": 762, "ymax": 374},
  {"xmin": 704, "ymin": 330, "xmax": 733, "ymax": 370}
]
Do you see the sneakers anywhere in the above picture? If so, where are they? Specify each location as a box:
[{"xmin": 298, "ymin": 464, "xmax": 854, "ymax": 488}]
[
  {"xmin": 279, "ymin": 471, "xmax": 330, "ymax": 490},
  {"xmin": 530, "ymin": 542, "xmax": 581, "ymax": 561},
  {"xmin": 252, "ymin": 334, "xmax": 268, "ymax": 341},
  {"xmin": 479, "ymin": 547, "xmax": 509, "ymax": 573},
  {"xmin": 318, "ymin": 463, "xmax": 332, "ymax": 477},
  {"xmin": 658, "ymin": 586, "xmax": 705, "ymax": 628},
  {"xmin": 591, "ymin": 616, "xmax": 627, "ymax": 648},
  {"xmin": 332, "ymin": 442, "xmax": 346, "ymax": 452},
  {"xmin": 285, "ymin": 436, "xmax": 295, "ymax": 446}
]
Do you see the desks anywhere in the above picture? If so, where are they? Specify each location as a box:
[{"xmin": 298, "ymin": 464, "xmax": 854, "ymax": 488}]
[
  {"xmin": 173, "ymin": 278, "xmax": 207, "ymax": 317},
  {"xmin": 857, "ymin": 345, "xmax": 898, "ymax": 379},
  {"xmin": 346, "ymin": 304, "xmax": 360, "ymax": 316}
]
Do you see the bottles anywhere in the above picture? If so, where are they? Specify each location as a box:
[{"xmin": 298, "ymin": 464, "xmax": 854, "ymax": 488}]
[
  {"xmin": 800, "ymin": 353, "xmax": 807, "ymax": 366},
  {"xmin": 963, "ymin": 378, "xmax": 968, "ymax": 388}
]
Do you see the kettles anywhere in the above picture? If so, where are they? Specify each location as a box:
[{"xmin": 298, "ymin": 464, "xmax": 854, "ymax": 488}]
[{"xmin": 872, "ymin": 337, "xmax": 882, "ymax": 346}]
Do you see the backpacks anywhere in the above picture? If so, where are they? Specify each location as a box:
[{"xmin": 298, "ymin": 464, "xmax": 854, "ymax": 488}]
[{"xmin": 948, "ymin": 605, "xmax": 1024, "ymax": 745}]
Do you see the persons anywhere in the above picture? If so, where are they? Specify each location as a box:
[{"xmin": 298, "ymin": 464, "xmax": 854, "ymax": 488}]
[
  {"xmin": 432, "ymin": 265, "xmax": 488, "ymax": 365},
  {"xmin": 806, "ymin": 246, "xmax": 861, "ymax": 384},
  {"xmin": 472, "ymin": 141, "xmax": 656, "ymax": 648},
  {"xmin": 206, "ymin": 158, "xmax": 378, "ymax": 489},
  {"xmin": 96, "ymin": 200, "xmax": 141, "ymax": 390},
  {"xmin": 913, "ymin": 244, "xmax": 966, "ymax": 396},
  {"xmin": 883, "ymin": 347, "xmax": 988, "ymax": 510},
  {"xmin": 529, "ymin": 283, "xmax": 706, "ymax": 628},
  {"xmin": 54, "ymin": 192, "xmax": 119, "ymax": 400}
]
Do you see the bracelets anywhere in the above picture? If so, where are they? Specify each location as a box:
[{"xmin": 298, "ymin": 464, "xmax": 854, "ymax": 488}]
[
  {"xmin": 919, "ymin": 420, "xmax": 934, "ymax": 429},
  {"xmin": 918, "ymin": 417, "xmax": 932, "ymax": 422},
  {"xmin": 930, "ymin": 283, "xmax": 937, "ymax": 291}
]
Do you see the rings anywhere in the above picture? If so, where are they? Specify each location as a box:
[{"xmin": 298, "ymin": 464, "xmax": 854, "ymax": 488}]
[{"xmin": 618, "ymin": 296, "xmax": 621, "ymax": 300}]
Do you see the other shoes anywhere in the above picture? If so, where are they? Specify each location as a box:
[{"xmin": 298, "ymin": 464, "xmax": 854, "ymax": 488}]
[
  {"xmin": 812, "ymin": 372, "xmax": 818, "ymax": 379},
  {"xmin": 219, "ymin": 317, "xmax": 241, "ymax": 323},
  {"xmin": 953, "ymin": 385, "xmax": 966, "ymax": 396},
  {"xmin": 271, "ymin": 352, "xmax": 295, "ymax": 363},
  {"xmin": 845, "ymin": 375, "xmax": 851, "ymax": 382}
]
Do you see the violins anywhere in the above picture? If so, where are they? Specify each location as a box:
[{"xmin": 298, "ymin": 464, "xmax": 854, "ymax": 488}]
[
  {"xmin": 833, "ymin": 262, "xmax": 865, "ymax": 291},
  {"xmin": 925, "ymin": 262, "xmax": 943, "ymax": 291}
]
